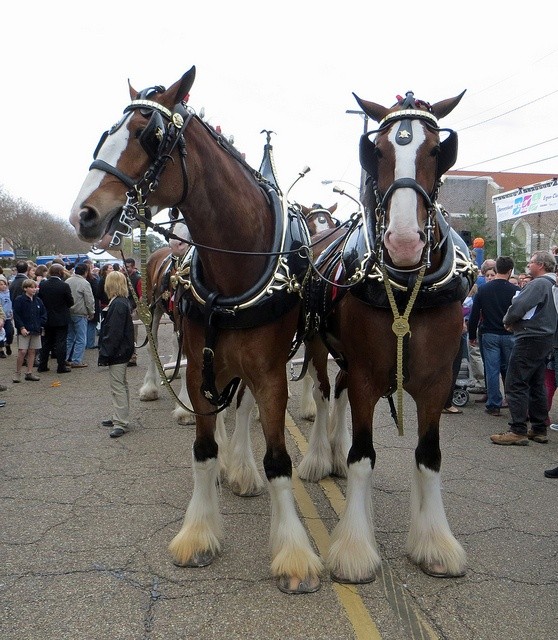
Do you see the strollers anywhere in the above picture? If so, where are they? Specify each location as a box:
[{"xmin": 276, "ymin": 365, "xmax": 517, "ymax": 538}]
[{"xmin": 452, "ymin": 332, "xmax": 487, "ymax": 407}]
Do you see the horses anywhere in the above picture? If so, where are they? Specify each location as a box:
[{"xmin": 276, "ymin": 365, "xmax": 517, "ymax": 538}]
[
  {"xmin": 69, "ymin": 64, "xmax": 327, "ymax": 594},
  {"xmin": 294, "ymin": 88, "xmax": 471, "ymax": 585}
]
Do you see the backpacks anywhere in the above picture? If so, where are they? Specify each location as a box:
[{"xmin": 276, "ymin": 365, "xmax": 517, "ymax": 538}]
[{"xmin": 536, "ymin": 275, "xmax": 558, "ymax": 315}]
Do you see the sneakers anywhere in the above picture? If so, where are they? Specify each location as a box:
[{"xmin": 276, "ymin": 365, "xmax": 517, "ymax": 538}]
[
  {"xmin": 527, "ymin": 429, "xmax": 548, "ymax": 443},
  {"xmin": 12, "ymin": 372, "xmax": 23, "ymax": 383},
  {"xmin": 24, "ymin": 372, "xmax": 40, "ymax": 381},
  {"xmin": 490, "ymin": 431, "xmax": 529, "ymax": 446}
]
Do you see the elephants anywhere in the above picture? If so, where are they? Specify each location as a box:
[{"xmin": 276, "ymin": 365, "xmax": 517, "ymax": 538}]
[
  {"xmin": 288, "ymin": 198, "xmax": 340, "ymax": 423},
  {"xmin": 139, "ymin": 210, "xmax": 210, "ymax": 426}
]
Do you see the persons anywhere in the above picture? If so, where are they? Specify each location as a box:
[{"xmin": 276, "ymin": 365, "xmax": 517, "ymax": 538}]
[
  {"xmin": 100, "ymin": 263, "xmax": 113, "ymax": 307},
  {"xmin": 124, "ymin": 259, "xmax": 141, "ymax": 367},
  {"xmin": 64, "ymin": 264, "xmax": 96, "ymax": 367},
  {"xmin": 96, "ymin": 272, "xmax": 132, "ymax": 438},
  {"xmin": 52, "ymin": 259, "xmax": 64, "ymax": 265},
  {"xmin": 522, "ymin": 278, "xmax": 531, "ymax": 287},
  {"xmin": 518, "ymin": 274, "xmax": 527, "ymax": 288},
  {"xmin": 83, "ymin": 260, "xmax": 93, "ymax": 267},
  {"xmin": 11, "ymin": 261, "xmax": 27, "ymax": 298},
  {"xmin": 0, "ymin": 384, "xmax": 9, "ymax": 392},
  {"xmin": 26, "ymin": 261, "xmax": 33, "ymax": 266},
  {"xmin": 0, "ymin": 399, "xmax": 7, "ymax": 408},
  {"xmin": 546, "ymin": 467, "xmax": 555, "ymax": 477},
  {"xmin": 542, "ymin": 361, "xmax": 558, "ymax": 434},
  {"xmin": 112, "ymin": 264, "xmax": 120, "ymax": 271},
  {"xmin": 508, "ymin": 276, "xmax": 517, "ymax": 285},
  {"xmin": 28, "ymin": 267, "xmax": 33, "ymax": 277},
  {"xmin": 491, "ymin": 250, "xmax": 557, "ymax": 446},
  {"xmin": 13, "ymin": 280, "xmax": 46, "ymax": 382},
  {"xmin": 34, "ymin": 266, "xmax": 47, "ymax": 290},
  {"xmin": 444, "ymin": 324, "xmax": 467, "ymax": 414},
  {"xmin": 0, "ymin": 304, "xmax": 8, "ymax": 359},
  {"xmin": 89, "ymin": 268, "xmax": 98, "ymax": 275},
  {"xmin": 1, "ymin": 274, "xmax": 14, "ymax": 359},
  {"xmin": 469, "ymin": 259, "xmax": 519, "ymax": 414},
  {"xmin": 483, "ymin": 268, "xmax": 495, "ymax": 283},
  {"xmin": 38, "ymin": 264, "xmax": 74, "ymax": 372}
]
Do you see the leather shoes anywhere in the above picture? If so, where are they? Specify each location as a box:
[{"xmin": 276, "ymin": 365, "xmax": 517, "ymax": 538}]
[
  {"xmin": 110, "ymin": 428, "xmax": 125, "ymax": 438},
  {"xmin": 71, "ymin": 362, "xmax": 88, "ymax": 368},
  {"xmin": 101, "ymin": 419, "xmax": 114, "ymax": 426},
  {"xmin": 38, "ymin": 368, "xmax": 50, "ymax": 373},
  {"xmin": 57, "ymin": 368, "xmax": 71, "ymax": 373},
  {"xmin": 441, "ymin": 406, "xmax": 462, "ymax": 414}
]
